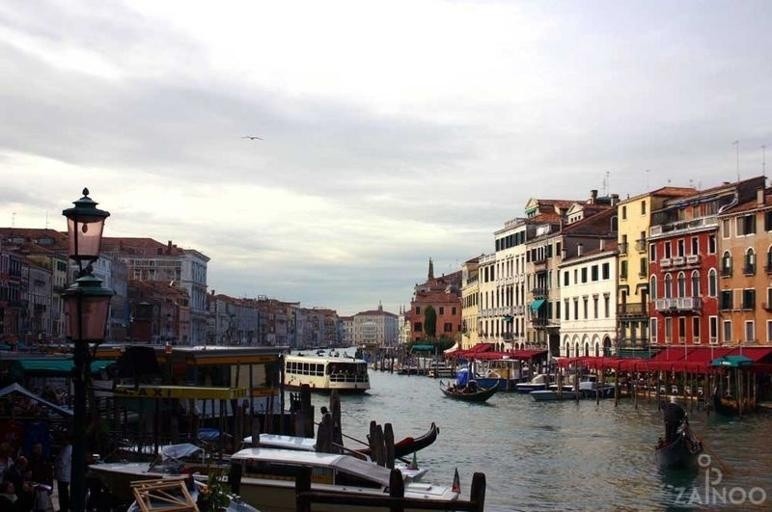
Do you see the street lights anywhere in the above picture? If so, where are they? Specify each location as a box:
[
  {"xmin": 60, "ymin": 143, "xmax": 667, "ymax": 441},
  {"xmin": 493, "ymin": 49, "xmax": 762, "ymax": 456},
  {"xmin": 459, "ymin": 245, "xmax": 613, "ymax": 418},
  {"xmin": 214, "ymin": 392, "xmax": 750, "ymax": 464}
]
[{"xmin": 52, "ymin": 187, "xmax": 117, "ymax": 512}]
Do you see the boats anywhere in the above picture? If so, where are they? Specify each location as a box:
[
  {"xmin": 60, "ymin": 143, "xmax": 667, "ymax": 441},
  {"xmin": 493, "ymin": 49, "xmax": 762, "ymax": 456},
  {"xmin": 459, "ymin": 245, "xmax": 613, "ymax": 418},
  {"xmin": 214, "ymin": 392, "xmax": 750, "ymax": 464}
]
[
  {"xmin": 368, "ymin": 346, "xmax": 615, "ymax": 403},
  {"xmin": 654, "ymin": 412, "xmax": 703, "ymax": 473}
]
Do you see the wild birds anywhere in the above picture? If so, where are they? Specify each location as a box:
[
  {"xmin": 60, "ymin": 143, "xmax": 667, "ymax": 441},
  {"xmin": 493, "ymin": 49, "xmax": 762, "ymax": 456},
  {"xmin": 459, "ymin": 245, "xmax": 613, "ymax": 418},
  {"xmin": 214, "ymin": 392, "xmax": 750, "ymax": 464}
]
[{"xmin": 241, "ymin": 136, "xmax": 263, "ymax": 141}]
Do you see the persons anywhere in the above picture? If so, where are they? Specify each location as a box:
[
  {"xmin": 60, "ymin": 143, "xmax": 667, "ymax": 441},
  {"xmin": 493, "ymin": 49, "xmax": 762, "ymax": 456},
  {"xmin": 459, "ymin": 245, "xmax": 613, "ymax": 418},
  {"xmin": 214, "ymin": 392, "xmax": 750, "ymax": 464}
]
[
  {"xmin": 0, "ymin": 431, "xmax": 73, "ymax": 512},
  {"xmin": 55, "ymin": 386, "xmax": 66, "ymax": 405},
  {"xmin": 41, "ymin": 386, "xmax": 59, "ymax": 404},
  {"xmin": 319, "ymin": 406, "xmax": 331, "ymax": 427},
  {"xmin": 659, "ymin": 393, "xmax": 686, "ymax": 445}
]
